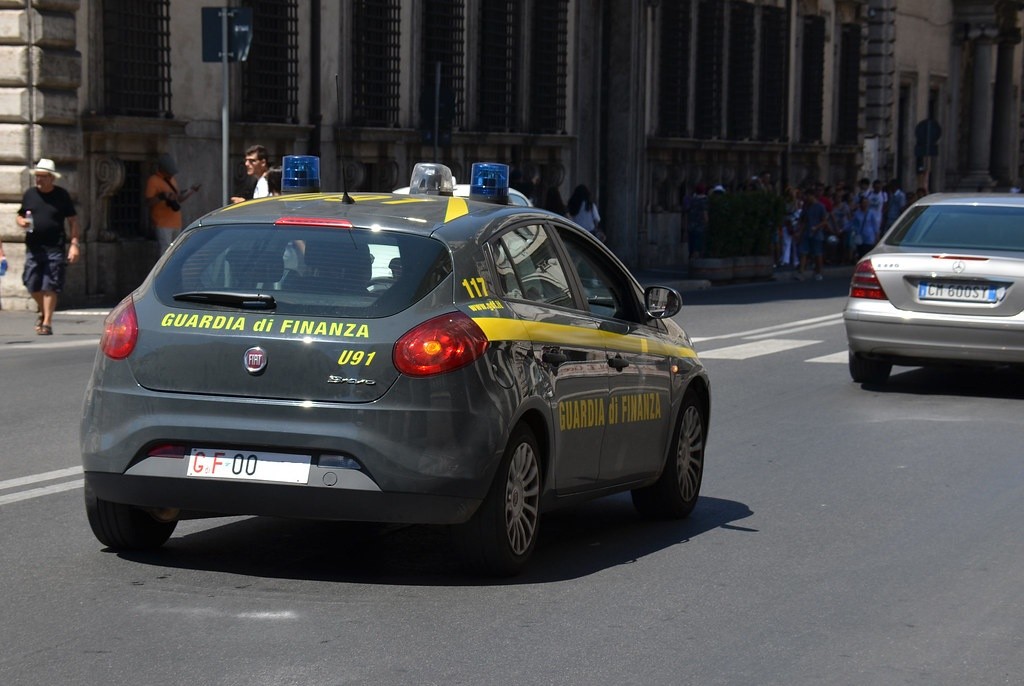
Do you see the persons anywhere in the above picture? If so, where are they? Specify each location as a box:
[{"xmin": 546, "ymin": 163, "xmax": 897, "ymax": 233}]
[
  {"xmin": 351, "ymin": 244, "xmax": 374, "ymax": 282},
  {"xmin": 682, "ymin": 170, "xmax": 927, "ymax": 280},
  {"xmin": 230, "ymin": 143, "xmax": 306, "ymax": 291},
  {"xmin": 15, "ymin": 158, "xmax": 80, "ymax": 335},
  {"xmin": 144, "ymin": 155, "xmax": 201, "ymax": 256},
  {"xmin": 659, "ymin": 291, "xmax": 678, "ymax": 318},
  {"xmin": 567, "ymin": 184, "xmax": 601, "ymax": 232}
]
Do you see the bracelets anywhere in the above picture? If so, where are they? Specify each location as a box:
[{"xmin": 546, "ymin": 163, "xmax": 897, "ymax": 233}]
[{"xmin": 69, "ymin": 242, "xmax": 80, "ymax": 248}]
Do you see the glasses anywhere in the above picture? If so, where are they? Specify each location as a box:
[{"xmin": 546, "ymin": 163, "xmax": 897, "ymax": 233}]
[{"xmin": 245, "ymin": 159, "xmax": 260, "ymax": 163}]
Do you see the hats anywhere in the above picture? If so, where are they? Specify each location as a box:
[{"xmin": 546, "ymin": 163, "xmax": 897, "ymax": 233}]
[
  {"xmin": 709, "ymin": 185, "xmax": 726, "ymax": 194},
  {"xmin": 28, "ymin": 159, "xmax": 62, "ymax": 179},
  {"xmin": 151, "ymin": 153, "xmax": 178, "ymax": 176}
]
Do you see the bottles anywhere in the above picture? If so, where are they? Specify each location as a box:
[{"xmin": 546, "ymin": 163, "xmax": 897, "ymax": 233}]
[{"xmin": 25, "ymin": 211, "xmax": 34, "ymax": 233}]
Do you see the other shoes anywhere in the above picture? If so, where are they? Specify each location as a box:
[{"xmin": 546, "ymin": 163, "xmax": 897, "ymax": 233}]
[{"xmin": 814, "ymin": 273, "xmax": 823, "ymax": 281}]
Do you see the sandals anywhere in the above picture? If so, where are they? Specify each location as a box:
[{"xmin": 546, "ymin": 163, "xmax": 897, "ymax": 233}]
[
  {"xmin": 33, "ymin": 313, "xmax": 44, "ymax": 331},
  {"xmin": 37, "ymin": 325, "xmax": 53, "ymax": 335}
]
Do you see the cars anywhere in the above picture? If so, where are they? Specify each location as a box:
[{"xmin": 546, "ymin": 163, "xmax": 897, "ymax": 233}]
[
  {"xmin": 840, "ymin": 192, "xmax": 1024, "ymax": 388},
  {"xmin": 75, "ymin": 152, "xmax": 714, "ymax": 575}
]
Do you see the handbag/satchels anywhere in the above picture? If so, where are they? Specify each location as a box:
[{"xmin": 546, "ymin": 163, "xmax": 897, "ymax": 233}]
[
  {"xmin": 853, "ymin": 233, "xmax": 863, "ymax": 245},
  {"xmin": 593, "ymin": 229, "xmax": 606, "ymax": 242}
]
